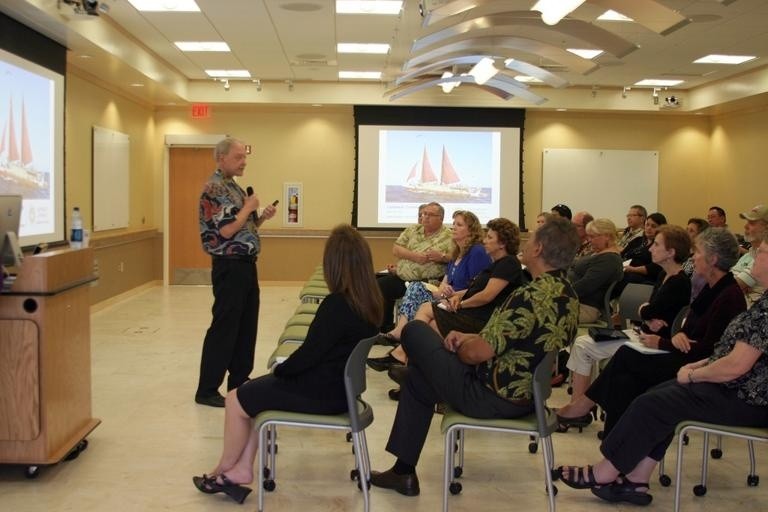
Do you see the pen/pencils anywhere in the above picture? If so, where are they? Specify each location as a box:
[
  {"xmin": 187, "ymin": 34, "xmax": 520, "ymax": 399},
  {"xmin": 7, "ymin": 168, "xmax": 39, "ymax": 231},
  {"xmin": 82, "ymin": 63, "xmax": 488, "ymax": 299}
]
[
  {"xmin": 445, "ymin": 296, "xmax": 451, "ymax": 305},
  {"xmin": 633, "ymin": 328, "xmax": 641, "ymax": 335}
]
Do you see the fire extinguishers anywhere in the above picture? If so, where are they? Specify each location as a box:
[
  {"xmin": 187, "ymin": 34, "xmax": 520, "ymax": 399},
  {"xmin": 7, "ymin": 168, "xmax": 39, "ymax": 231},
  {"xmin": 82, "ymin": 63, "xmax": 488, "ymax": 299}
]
[{"xmin": 289, "ymin": 193, "xmax": 298, "ymax": 223}]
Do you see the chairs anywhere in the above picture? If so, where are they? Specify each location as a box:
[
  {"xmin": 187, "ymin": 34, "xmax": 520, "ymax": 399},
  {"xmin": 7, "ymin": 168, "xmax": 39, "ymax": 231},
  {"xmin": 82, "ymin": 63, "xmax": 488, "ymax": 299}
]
[
  {"xmin": 441, "ymin": 351, "xmax": 559, "ymax": 512},
  {"xmin": 674, "ymin": 421, "xmax": 768, "ymax": 512},
  {"xmin": 294, "ymin": 303, "xmax": 321, "ymax": 314},
  {"xmin": 298, "ymin": 262, "xmax": 330, "ymax": 303},
  {"xmin": 278, "ymin": 326, "xmax": 309, "ymax": 348},
  {"xmin": 255, "ymin": 335, "xmax": 379, "ymax": 511},
  {"xmin": 285, "ymin": 314, "xmax": 315, "ymax": 326},
  {"xmin": 618, "ymin": 282, "xmax": 653, "ymax": 327},
  {"xmin": 567, "ymin": 280, "xmax": 617, "ymax": 395},
  {"xmin": 267, "ymin": 341, "xmax": 304, "ymax": 368}
]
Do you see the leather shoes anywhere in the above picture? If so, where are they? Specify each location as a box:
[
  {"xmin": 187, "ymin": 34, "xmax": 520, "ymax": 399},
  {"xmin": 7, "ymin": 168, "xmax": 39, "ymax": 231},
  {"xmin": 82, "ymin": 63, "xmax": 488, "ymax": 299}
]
[
  {"xmin": 366, "ymin": 332, "xmax": 407, "ymax": 401},
  {"xmin": 370, "ymin": 469, "xmax": 420, "ymax": 496},
  {"xmin": 195, "ymin": 392, "xmax": 225, "ymax": 407}
]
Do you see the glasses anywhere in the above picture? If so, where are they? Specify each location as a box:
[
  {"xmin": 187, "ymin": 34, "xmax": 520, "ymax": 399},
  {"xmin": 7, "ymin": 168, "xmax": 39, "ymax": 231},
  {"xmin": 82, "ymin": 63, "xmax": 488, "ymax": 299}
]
[{"xmin": 421, "ymin": 211, "xmax": 441, "ymax": 218}]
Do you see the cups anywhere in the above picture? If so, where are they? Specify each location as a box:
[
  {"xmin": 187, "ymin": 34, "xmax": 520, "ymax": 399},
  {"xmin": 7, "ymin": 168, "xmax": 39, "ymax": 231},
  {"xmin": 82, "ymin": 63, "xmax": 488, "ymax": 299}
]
[{"xmin": 83, "ymin": 229, "xmax": 90, "ymax": 249}]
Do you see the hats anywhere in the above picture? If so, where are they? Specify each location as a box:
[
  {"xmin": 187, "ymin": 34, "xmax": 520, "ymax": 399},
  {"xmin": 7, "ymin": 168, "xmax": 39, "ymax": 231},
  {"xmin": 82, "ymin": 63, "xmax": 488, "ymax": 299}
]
[{"xmin": 740, "ymin": 206, "xmax": 768, "ymax": 223}]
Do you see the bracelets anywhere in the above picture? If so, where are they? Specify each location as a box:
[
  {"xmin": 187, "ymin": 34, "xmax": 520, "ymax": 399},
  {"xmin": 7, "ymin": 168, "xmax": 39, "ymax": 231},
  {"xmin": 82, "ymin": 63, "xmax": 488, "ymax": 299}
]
[
  {"xmin": 234, "ymin": 215, "xmax": 243, "ymax": 228},
  {"xmin": 459, "ymin": 300, "xmax": 463, "ymax": 310},
  {"xmin": 688, "ymin": 368, "xmax": 694, "ymax": 384}
]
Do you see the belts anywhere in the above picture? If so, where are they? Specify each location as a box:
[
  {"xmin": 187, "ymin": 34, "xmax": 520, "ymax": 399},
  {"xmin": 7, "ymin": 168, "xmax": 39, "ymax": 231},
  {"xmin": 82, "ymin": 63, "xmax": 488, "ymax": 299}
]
[{"xmin": 212, "ymin": 254, "xmax": 259, "ymax": 262}]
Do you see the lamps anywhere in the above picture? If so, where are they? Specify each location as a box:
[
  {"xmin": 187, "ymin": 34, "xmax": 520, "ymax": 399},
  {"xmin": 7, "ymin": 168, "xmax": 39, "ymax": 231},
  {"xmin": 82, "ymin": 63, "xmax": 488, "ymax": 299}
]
[
  {"xmin": 619, "ymin": 86, "xmax": 629, "ymax": 97},
  {"xmin": 652, "ymin": 88, "xmax": 661, "ymax": 106},
  {"xmin": 288, "ymin": 81, "xmax": 297, "ymax": 95},
  {"xmin": 438, "ymin": 71, "xmax": 464, "ymax": 93},
  {"xmin": 222, "ymin": 81, "xmax": 231, "ymax": 91},
  {"xmin": 255, "ymin": 81, "xmax": 264, "ymax": 94},
  {"xmin": 530, "ymin": 0, "xmax": 587, "ymax": 26},
  {"xmin": 591, "ymin": 85, "xmax": 596, "ymax": 97},
  {"xmin": 468, "ymin": 57, "xmax": 498, "ymax": 87}
]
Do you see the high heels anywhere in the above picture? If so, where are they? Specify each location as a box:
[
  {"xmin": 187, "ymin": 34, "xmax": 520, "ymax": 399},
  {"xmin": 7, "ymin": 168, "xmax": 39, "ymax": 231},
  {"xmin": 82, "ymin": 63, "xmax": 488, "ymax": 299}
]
[
  {"xmin": 551, "ymin": 405, "xmax": 597, "ymax": 433},
  {"xmin": 193, "ymin": 475, "xmax": 252, "ymax": 503}
]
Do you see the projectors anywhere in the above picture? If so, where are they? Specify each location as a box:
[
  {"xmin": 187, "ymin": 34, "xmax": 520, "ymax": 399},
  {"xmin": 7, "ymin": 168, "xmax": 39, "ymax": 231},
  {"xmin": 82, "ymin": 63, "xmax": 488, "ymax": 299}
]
[{"xmin": 419, "ymin": 0, "xmax": 454, "ymax": 17}]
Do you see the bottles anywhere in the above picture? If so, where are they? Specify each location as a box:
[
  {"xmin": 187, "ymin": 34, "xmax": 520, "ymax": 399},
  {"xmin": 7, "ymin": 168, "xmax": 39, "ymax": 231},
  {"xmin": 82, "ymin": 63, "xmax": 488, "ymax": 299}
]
[{"xmin": 70, "ymin": 207, "xmax": 83, "ymax": 249}]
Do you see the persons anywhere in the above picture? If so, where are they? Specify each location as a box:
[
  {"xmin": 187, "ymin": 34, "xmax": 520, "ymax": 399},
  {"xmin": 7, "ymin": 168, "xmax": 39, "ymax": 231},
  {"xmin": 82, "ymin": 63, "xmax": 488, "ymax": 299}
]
[
  {"xmin": 196, "ymin": 138, "xmax": 277, "ymax": 407},
  {"xmin": 555, "ymin": 227, "xmax": 747, "ymax": 426},
  {"xmin": 369, "ymin": 218, "xmax": 579, "ymax": 496},
  {"xmin": 193, "ymin": 224, "xmax": 383, "ymax": 503},
  {"xmin": 557, "ymin": 230, "xmax": 768, "ymax": 506},
  {"xmin": 619, "ymin": 206, "xmax": 768, "ymax": 302},
  {"xmin": 536, "ymin": 203, "xmax": 624, "ymax": 318},
  {"xmin": 556, "ymin": 226, "xmax": 692, "ymax": 433},
  {"xmin": 373, "ymin": 203, "xmax": 491, "ymax": 345},
  {"xmin": 366, "ymin": 217, "xmax": 522, "ymax": 400}
]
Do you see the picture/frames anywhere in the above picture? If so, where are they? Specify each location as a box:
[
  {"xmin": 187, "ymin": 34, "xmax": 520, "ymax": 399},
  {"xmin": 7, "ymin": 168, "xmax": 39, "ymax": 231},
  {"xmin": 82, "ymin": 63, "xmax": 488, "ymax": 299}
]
[{"xmin": 282, "ymin": 182, "xmax": 304, "ymax": 228}]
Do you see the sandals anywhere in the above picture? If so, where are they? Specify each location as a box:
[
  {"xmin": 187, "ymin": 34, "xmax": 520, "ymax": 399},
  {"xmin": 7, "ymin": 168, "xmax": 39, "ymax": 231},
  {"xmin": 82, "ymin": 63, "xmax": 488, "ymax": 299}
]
[
  {"xmin": 558, "ymin": 465, "xmax": 615, "ymax": 488},
  {"xmin": 591, "ymin": 474, "xmax": 653, "ymax": 506}
]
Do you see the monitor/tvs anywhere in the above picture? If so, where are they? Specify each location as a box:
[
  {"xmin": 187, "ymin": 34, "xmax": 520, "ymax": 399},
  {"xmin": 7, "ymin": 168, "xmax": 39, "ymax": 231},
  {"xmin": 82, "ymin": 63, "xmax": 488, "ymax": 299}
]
[{"xmin": 1, "ymin": 195, "xmax": 22, "ymax": 270}]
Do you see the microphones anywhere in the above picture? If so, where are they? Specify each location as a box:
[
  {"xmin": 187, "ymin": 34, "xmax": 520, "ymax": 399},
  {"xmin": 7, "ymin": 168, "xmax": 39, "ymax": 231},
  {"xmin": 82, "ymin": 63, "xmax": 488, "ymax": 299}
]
[{"xmin": 246, "ymin": 186, "xmax": 260, "ymax": 223}]
